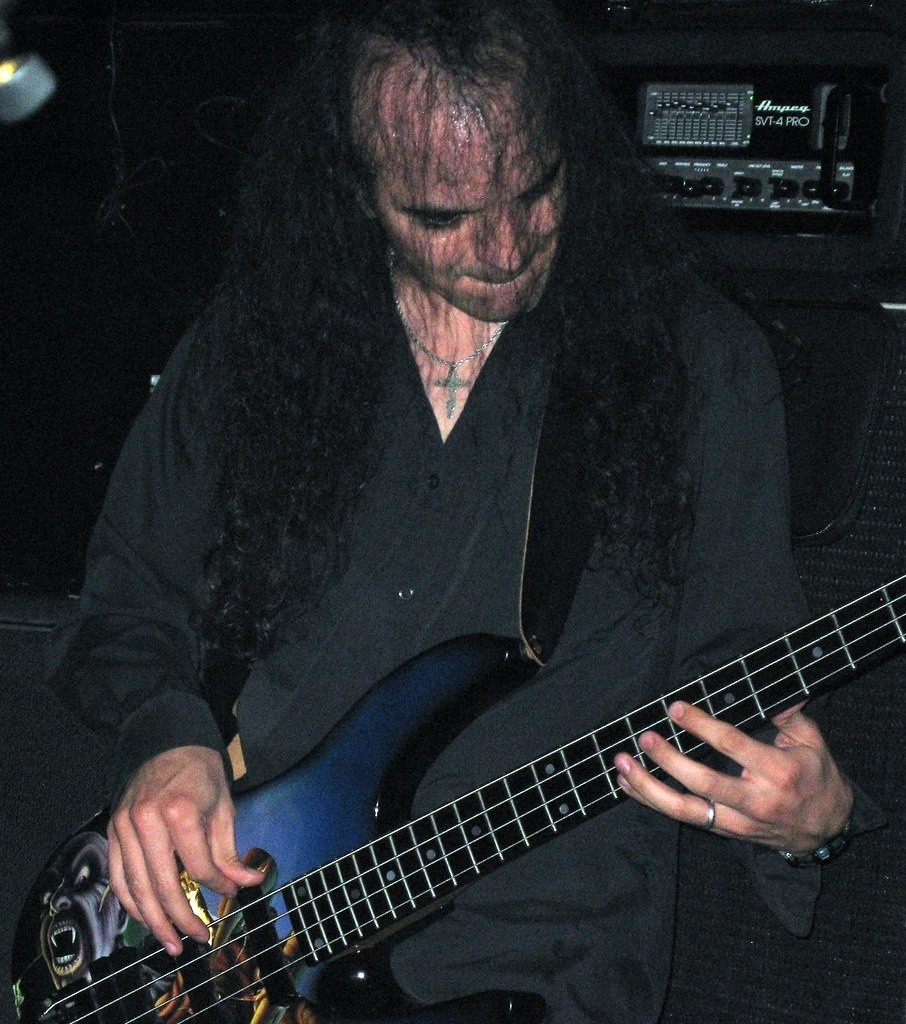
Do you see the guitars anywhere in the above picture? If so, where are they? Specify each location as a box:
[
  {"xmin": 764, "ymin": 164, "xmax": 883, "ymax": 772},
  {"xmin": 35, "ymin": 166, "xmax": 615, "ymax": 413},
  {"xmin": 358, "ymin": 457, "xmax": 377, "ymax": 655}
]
[{"xmin": 8, "ymin": 566, "xmax": 906, "ymax": 1024}]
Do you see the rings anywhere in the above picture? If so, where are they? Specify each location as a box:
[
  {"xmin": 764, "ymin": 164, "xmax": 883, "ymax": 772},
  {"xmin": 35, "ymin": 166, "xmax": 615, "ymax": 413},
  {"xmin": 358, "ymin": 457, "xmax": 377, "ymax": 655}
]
[{"xmin": 700, "ymin": 800, "xmax": 716, "ymax": 831}]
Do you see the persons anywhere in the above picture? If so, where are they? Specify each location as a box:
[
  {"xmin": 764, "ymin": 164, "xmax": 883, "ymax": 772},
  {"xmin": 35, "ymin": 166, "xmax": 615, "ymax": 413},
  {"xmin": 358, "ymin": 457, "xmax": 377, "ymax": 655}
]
[{"xmin": 49, "ymin": 0, "xmax": 885, "ymax": 1024}]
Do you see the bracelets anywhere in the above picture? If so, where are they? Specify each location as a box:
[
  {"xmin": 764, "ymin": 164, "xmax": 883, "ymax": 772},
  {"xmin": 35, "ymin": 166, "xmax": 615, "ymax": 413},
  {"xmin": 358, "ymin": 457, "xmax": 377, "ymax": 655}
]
[{"xmin": 777, "ymin": 818, "xmax": 853, "ymax": 866}]
